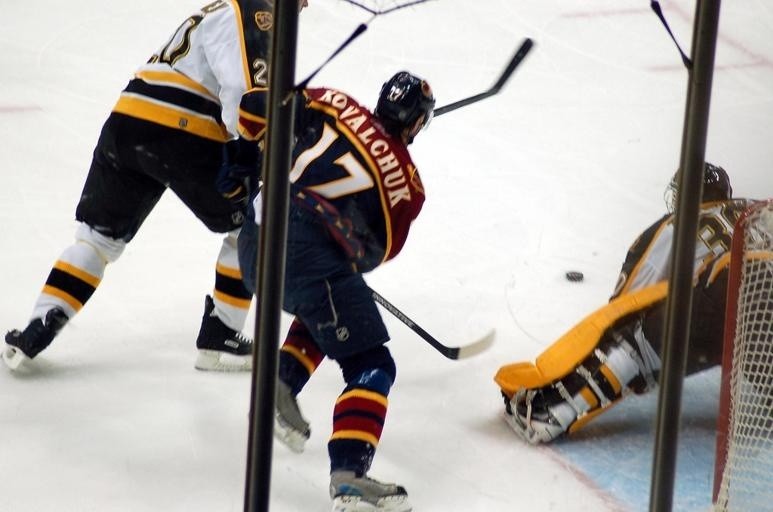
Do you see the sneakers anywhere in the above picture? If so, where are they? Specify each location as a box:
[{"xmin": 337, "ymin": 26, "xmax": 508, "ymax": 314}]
[
  {"xmin": 329, "ymin": 470, "xmax": 407, "ymax": 498},
  {"xmin": 196, "ymin": 317, "xmax": 254, "ymax": 356},
  {"xmin": 506, "ymin": 398, "xmax": 574, "ymax": 444},
  {"xmin": 275, "ymin": 374, "xmax": 310, "ymax": 440},
  {"xmin": 5, "ymin": 324, "xmax": 52, "ymax": 359}
]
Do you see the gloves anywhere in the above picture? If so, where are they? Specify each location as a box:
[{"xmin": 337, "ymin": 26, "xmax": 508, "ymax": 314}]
[{"xmin": 214, "ymin": 140, "xmax": 263, "ymax": 204}]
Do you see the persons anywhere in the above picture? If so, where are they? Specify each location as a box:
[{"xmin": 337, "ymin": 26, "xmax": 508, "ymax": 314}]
[
  {"xmin": 492, "ymin": 154, "xmax": 772, "ymax": 436},
  {"xmin": 1, "ymin": 0, "xmax": 310, "ymax": 360},
  {"xmin": 233, "ymin": 69, "xmax": 437, "ymax": 502}
]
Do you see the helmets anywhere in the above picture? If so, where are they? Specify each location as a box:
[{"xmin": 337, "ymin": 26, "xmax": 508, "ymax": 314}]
[
  {"xmin": 377, "ymin": 72, "xmax": 435, "ymax": 147},
  {"xmin": 665, "ymin": 163, "xmax": 731, "ymax": 213}
]
